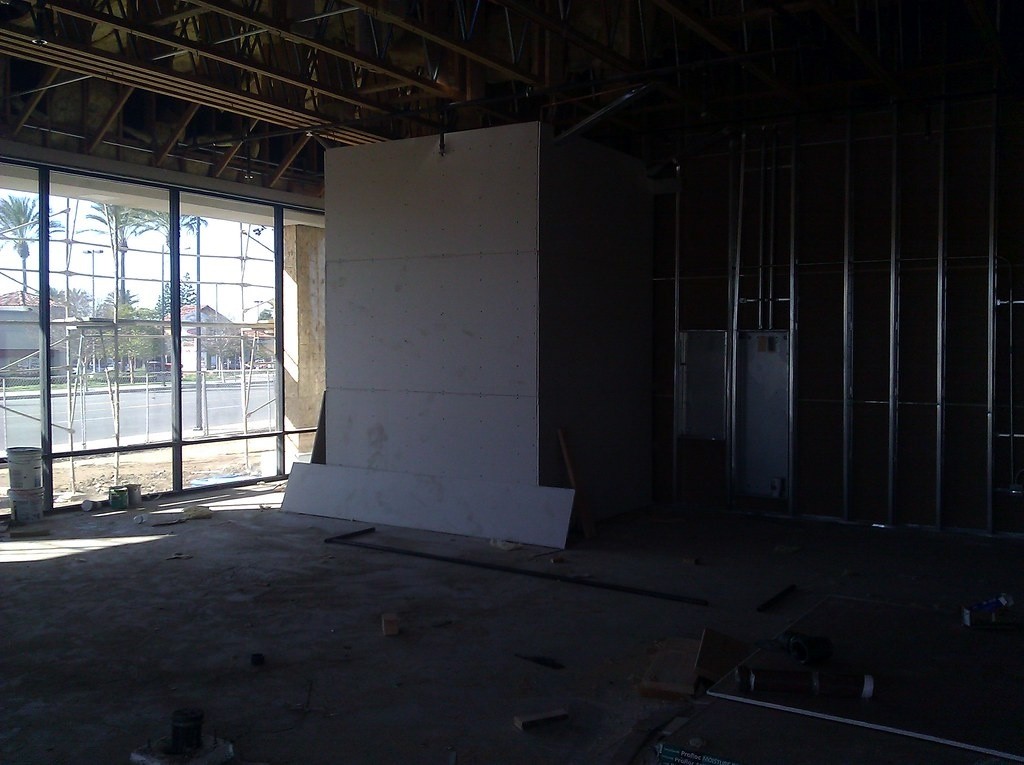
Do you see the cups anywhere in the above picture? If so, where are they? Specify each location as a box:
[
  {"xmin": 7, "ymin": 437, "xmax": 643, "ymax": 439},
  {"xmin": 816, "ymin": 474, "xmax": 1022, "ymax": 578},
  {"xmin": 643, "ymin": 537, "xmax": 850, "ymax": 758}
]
[
  {"xmin": 171, "ymin": 707, "xmax": 204, "ymax": 753},
  {"xmin": 81, "ymin": 500, "xmax": 102, "ymax": 511},
  {"xmin": 789, "ymin": 635, "xmax": 834, "ymax": 664}
]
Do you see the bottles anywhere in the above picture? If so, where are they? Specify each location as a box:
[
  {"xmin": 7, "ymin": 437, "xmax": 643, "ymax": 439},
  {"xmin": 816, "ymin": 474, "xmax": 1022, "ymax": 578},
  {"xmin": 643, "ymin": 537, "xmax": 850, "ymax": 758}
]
[{"xmin": 134, "ymin": 514, "xmax": 147, "ymax": 523}]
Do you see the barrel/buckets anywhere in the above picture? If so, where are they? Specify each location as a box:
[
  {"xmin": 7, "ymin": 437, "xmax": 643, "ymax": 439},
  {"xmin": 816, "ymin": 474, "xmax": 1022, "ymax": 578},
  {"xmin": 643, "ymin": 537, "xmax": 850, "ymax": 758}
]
[
  {"xmin": 109, "ymin": 484, "xmax": 141, "ymax": 510},
  {"xmin": 7, "ymin": 447, "xmax": 42, "ymax": 489},
  {"xmin": 7, "ymin": 489, "xmax": 44, "ymax": 524}
]
